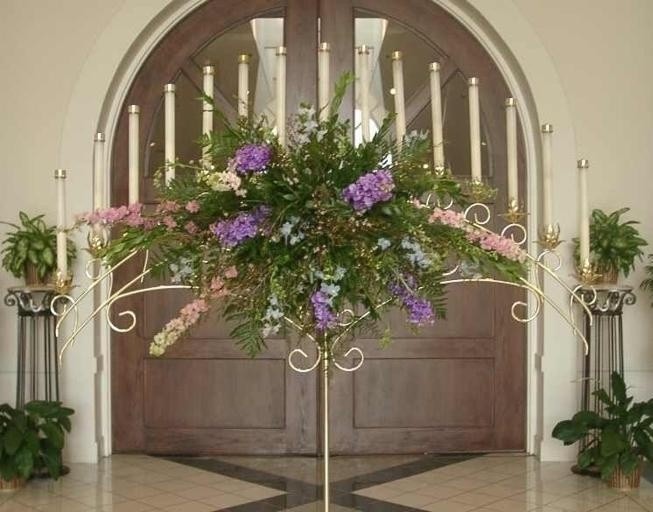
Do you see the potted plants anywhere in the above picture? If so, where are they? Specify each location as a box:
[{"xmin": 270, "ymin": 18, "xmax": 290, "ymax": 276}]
[
  {"xmin": 571, "ymin": 207, "xmax": 648, "ymax": 285},
  {"xmin": 0, "ymin": 400, "xmax": 75, "ymax": 491},
  {"xmin": 552, "ymin": 371, "xmax": 653, "ymax": 490},
  {"xmin": 0, "ymin": 211, "xmax": 76, "ymax": 287}
]
